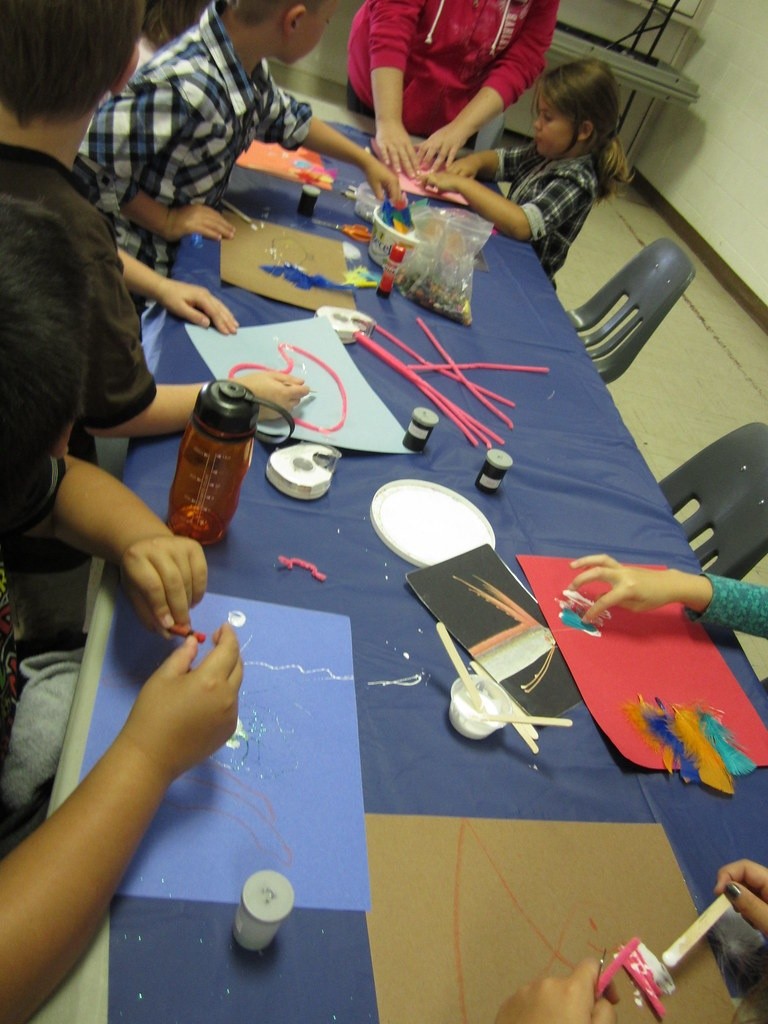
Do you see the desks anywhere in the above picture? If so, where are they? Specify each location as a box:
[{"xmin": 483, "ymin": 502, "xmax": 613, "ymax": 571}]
[{"xmin": 33, "ymin": 118, "xmax": 768, "ymax": 1024}]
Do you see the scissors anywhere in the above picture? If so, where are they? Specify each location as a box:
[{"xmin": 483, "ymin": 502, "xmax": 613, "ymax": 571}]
[{"xmin": 312, "ymin": 219, "xmax": 372, "ymax": 243}]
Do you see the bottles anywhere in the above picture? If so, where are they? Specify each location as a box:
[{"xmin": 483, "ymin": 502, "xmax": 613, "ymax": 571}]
[
  {"xmin": 475, "ymin": 449, "xmax": 513, "ymax": 494},
  {"xmin": 233, "ymin": 869, "xmax": 295, "ymax": 950},
  {"xmin": 403, "ymin": 407, "xmax": 439, "ymax": 452},
  {"xmin": 166, "ymin": 380, "xmax": 294, "ymax": 546},
  {"xmin": 298, "ymin": 184, "xmax": 321, "ymax": 215}
]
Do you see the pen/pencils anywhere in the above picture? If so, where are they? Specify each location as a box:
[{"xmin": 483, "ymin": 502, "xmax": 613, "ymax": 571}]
[
  {"xmin": 284, "ymin": 382, "xmax": 318, "ymax": 393},
  {"xmin": 170, "ymin": 625, "xmax": 205, "ymax": 643},
  {"xmin": 221, "ymin": 198, "xmax": 252, "ymax": 223},
  {"xmin": 341, "ymin": 191, "xmax": 356, "ymax": 200}
]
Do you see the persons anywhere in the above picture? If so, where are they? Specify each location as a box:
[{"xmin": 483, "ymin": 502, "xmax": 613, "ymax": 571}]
[
  {"xmin": 570, "ymin": 553, "xmax": 768, "ymax": 642},
  {"xmin": 0, "ymin": 0, "xmax": 309, "ymax": 467},
  {"xmin": 76, "ymin": 1, "xmax": 400, "ymax": 282},
  {"xmin": 0, "ymin": 193, "xmax": 244, "ymax": 1024},
  {"xmin": 498, "ymin": 858, "xmax": 768, "ymax": 1023},
  {"xmin": 417, "ymin": 59, "xmax": 631, "ymax": 288},
  {"xmin": 345, "ymin": 0, "xmax": 561, "ymax": 180}
]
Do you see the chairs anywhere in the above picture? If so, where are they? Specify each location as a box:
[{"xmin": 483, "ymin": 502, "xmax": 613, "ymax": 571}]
[
  {"xmin": 565, "ymin": 235, "xmax": 696, "ymax": 385},
  {"xmin": 656, "ymin": 422, "xmax": 766, "ymax": 584}
]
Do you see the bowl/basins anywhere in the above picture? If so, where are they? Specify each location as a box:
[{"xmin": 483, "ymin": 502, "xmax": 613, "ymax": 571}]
[
  {"xmin": 367, "ymin": 204, "xmax": 421, "ymax": 270},
  {"xmin": 449, "ymin": 674, "xmax": 514, "ymax": 741}
]
